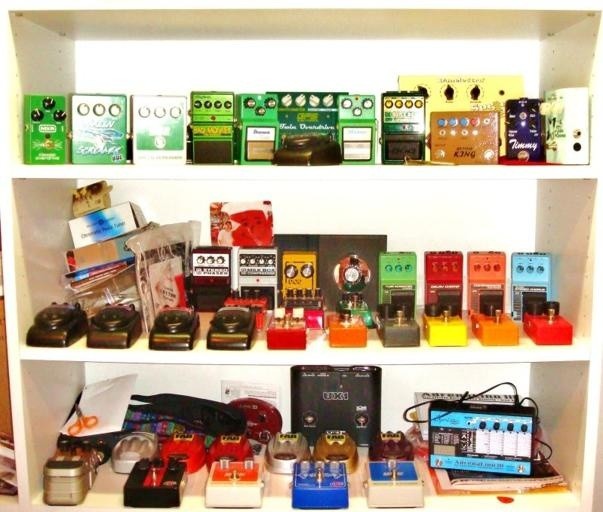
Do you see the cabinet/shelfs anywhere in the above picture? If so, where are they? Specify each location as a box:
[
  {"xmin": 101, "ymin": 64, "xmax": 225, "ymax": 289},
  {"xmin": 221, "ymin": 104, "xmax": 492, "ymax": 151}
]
[{"xmin": 0, "ymin": 10, "xmax": 603, "ymax": 512}]
